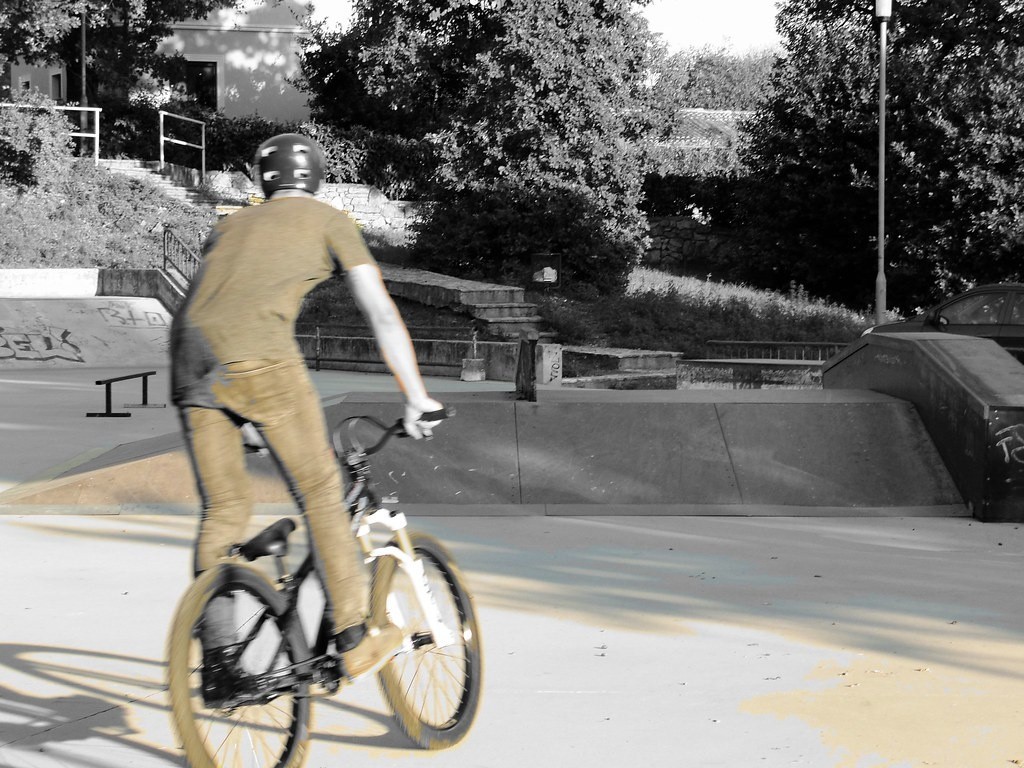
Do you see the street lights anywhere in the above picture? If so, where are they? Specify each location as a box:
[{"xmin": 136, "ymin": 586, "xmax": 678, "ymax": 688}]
[{"xmin": 875, "ymin": 0, "xmax": 893, "ymax": 325}]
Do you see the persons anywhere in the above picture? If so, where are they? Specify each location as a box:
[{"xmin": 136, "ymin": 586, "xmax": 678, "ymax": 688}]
[{"xmin": 169, "ymin": 132, "xmax": 445, "ymax": 705}]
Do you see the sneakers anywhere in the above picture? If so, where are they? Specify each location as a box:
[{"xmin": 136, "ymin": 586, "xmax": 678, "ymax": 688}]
[
  {"xmin": 339, "ymin": 623, "xmax": 406, "ymax": 685},
  {"xmin": 199, "ymin": 661, "xmax": 280, "ymax": 709}
]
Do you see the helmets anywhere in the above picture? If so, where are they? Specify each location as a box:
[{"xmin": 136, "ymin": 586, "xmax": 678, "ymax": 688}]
[{"xmin": 256, "ymin": 133, "xmax": 324, "ymax": 199}]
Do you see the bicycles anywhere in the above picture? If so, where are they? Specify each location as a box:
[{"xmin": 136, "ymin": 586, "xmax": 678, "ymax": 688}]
[{"xmin": 168, "ymin": 404, "xmax": 483, "ymax": 768}]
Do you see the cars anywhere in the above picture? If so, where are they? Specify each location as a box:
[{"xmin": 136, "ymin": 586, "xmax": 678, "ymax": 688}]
[{"xmin": 860, "ymin": 280, "xmax": 1024, "ymax": 363}]
